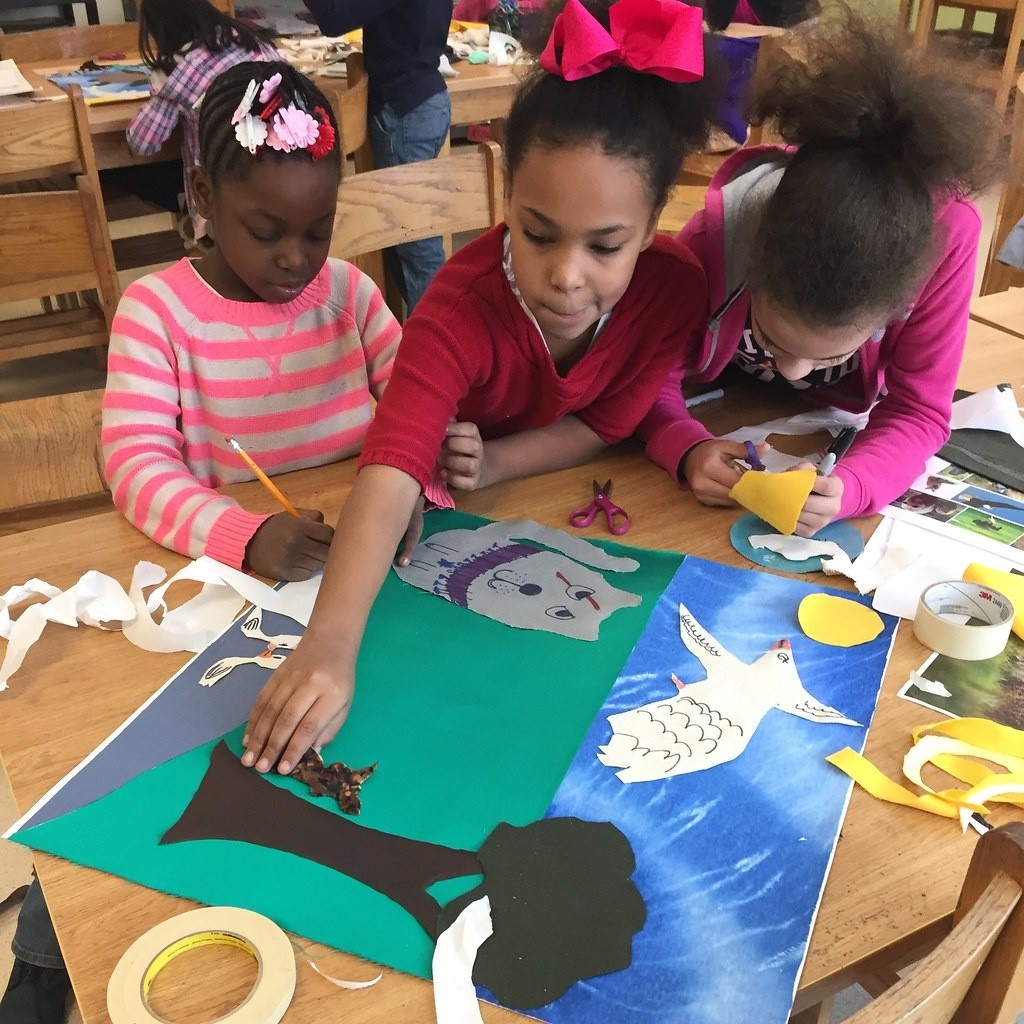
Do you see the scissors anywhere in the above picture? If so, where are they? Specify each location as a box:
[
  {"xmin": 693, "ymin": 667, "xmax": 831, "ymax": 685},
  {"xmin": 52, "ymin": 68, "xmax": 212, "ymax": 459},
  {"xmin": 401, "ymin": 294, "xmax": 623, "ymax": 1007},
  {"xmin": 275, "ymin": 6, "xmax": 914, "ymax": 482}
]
[
  {"xmin": 570, "ymin": 477, "xmax": 634, "ymax": 536},
  {"xmin": 731, "ymin": 440, "xmax": 771, "ymax": 481}
]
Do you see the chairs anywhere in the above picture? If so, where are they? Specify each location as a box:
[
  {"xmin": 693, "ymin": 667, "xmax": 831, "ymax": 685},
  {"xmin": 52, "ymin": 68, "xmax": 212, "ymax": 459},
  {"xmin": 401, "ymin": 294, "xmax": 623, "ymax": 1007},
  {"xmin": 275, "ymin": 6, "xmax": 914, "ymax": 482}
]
[
  {"xmin": 328, "ymin": 51, "xmax": 371, "ymax": 177},
  {"xmin": 328, "ymin": 141, "xmax": 503, "ymax": 257},
  {"xmin": 0, "ymin": 83, "xmax": 120, "ymax": 294},
  {"xmin": 0, "ymin": 22, "xmax": 156, "ymax": 68},
  {"xmin": 828, "ymin": 823, "xmax": 1024, "ymax": 1024},
  {"xmin": 0, "ymin": 177, "xmax": 117, "ymax": 536}
]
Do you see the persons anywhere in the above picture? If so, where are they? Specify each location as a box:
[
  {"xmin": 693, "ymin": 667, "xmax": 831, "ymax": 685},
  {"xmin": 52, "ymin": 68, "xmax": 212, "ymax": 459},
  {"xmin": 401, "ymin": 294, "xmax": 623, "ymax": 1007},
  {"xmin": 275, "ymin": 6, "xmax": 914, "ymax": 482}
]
[
  {"xmin": 240, "ymin": 0, "xmax": 728, "ymax": 775},
  {"xmin": 126, "ymin": 0, "xmax": 289, "ymax": 245},
  {"xmin": 101, "ymin": 57, "xmax": 455, "ymax": 582},
  {"xmin": 631, "ymin": 8, "xmax": 1018, "ymax": 538},
  {"xmin": 303, "ymin": 0, "xmax": 454, "ymax": 320}
]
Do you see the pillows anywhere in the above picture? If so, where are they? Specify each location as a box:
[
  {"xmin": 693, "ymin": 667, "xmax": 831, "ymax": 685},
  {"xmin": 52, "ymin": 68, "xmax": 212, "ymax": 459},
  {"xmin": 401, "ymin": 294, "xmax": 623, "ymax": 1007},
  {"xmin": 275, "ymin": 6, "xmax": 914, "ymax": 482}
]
[{"xmin": 701, "ymin": 32, "xmax": 760, "ymax": 145}]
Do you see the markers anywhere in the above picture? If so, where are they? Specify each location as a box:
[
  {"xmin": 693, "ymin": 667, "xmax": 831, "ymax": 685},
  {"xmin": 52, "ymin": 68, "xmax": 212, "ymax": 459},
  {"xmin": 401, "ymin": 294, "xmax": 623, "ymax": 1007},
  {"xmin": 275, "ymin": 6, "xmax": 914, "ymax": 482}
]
[
  {"xmin": 816, "ymin": 425, "xmax": 858, "ymax": 477},
  {"xmin": 684, "ymin": 387, "xmax": 729, "ymax": 411}
]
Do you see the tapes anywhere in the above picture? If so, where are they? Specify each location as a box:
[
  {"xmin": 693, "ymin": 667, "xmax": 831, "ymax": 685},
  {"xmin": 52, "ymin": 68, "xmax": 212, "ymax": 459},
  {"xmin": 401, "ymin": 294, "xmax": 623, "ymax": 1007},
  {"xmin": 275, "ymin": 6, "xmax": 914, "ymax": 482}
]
[
  {"xmin": 106, "ymin": 904, "xmax": 305, "ymax": 1024},
  {"xmin": 912, "ymin": 580, "xmax": 1014, "ymax": 661}
]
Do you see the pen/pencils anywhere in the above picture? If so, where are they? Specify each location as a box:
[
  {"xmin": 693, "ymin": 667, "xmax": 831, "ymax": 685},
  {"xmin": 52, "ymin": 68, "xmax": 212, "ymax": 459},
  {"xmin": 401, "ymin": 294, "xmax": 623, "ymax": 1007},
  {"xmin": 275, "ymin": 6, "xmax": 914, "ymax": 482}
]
[{"xmin": 219, "ymin": 435, "xmax": 306, "ymax": 523}]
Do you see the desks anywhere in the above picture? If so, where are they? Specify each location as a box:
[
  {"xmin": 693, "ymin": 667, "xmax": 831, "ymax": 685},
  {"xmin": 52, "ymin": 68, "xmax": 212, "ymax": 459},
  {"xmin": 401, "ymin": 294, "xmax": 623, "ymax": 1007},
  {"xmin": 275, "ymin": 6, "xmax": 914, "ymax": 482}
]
[{"xmin": 0, "ymin": 0, "xmax": 1024, "ymax": 1024}]
